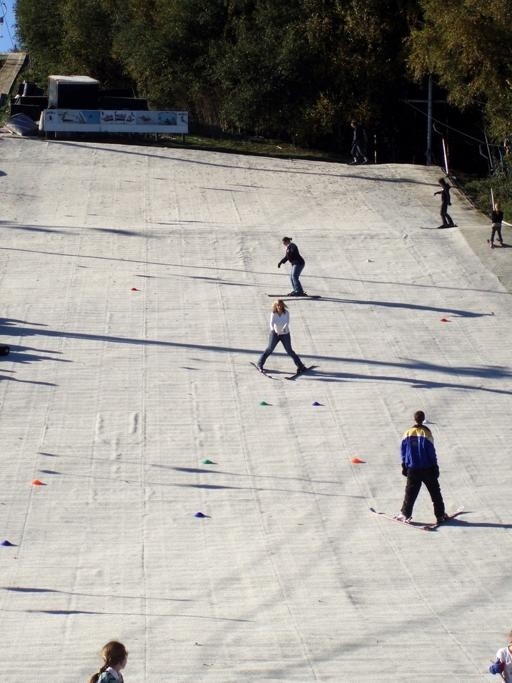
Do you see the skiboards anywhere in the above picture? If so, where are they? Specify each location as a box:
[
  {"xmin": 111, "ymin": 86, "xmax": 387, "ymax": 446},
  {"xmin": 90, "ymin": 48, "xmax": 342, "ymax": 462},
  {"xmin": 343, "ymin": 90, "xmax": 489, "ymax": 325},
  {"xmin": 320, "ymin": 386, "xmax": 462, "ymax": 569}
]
[
  {"xmin": 267, "ymin": 292, "xmax": 320, "ymax": 302},
  {"xmin": 369, "ymin": 504, "xmax": 468, "ymax": 530},
  {"xmin": 419, "ymin": 224, "xmax": 458, "ymax": 228},
  {"xmin": 248, "ymin": 360, "xmax": 318, "ymax": 380}
]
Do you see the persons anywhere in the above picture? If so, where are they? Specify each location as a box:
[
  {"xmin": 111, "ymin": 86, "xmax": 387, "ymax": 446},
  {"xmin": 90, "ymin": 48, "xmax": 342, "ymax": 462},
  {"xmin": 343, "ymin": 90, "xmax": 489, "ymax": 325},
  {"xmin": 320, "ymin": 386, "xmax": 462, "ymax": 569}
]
[
  {"xmin": 276, "ymin": 237, "xmax": 305, "ymax": 295},
  {"xmin": 489, "ymin": 629, "xmax": 512, "ymax": 683},
  {"xmin": 490, "ymin": 202, "xmax": 504, "ymax": 248},
  {"xmin": 88, "ymin": 641, "xmax": 128, "ymax": 683},
  {"xmin": 433, "ymin": 178, "xmax": 454, "ymax": 228},
  {"xmin": 349, "ymin": 121, "xmax": 369, "ymax": 165},
  {"xmin": 254, "ymin": 300, "xmax": 306, "ymax": 375},
  {"xmin": 399, "ymin": 411, "xmax": 448, "ymax": 525}
]
